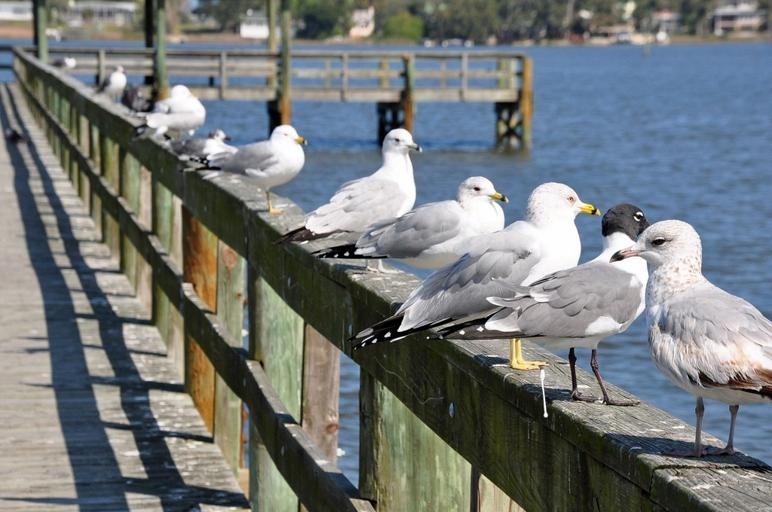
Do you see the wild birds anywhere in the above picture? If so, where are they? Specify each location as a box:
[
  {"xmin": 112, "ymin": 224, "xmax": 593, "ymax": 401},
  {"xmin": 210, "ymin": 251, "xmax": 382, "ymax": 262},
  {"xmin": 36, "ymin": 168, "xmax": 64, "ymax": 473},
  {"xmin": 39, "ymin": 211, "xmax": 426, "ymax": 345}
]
[
  {"xmin": 178, "ymin": 124, "xmax": 308, "ymax": 214},
  {"xmin": 607, "ymin": 218, "xmax": 772, "ymax": 458},
  {"xmin": 128, "ymin": 84, "xmax": 207, "ymax": 142},
  {"xmin": 307, "ymin": 175, "xmax": 509, "ymax": 270},
  {"xmin": 158, "ymin": 126, "xmax": 236, "ymax": 163},
  {"xmin": 92, "ymin": 66, "xmax": 128, "ymax": 104},
  {"xmin": 270, "ymin": 128, "xmax": 423, "ymax": 247},
  {"xmin": 121, "ymin": 75, "xmax": 158, "ymax": 118},
  {"xmin": 344, "ymin": 181, "xmax": 600, "ymax": 371},
  {"xmin": 423, "ymin": 202, "xmax": 651, "ymax": 406}
]
